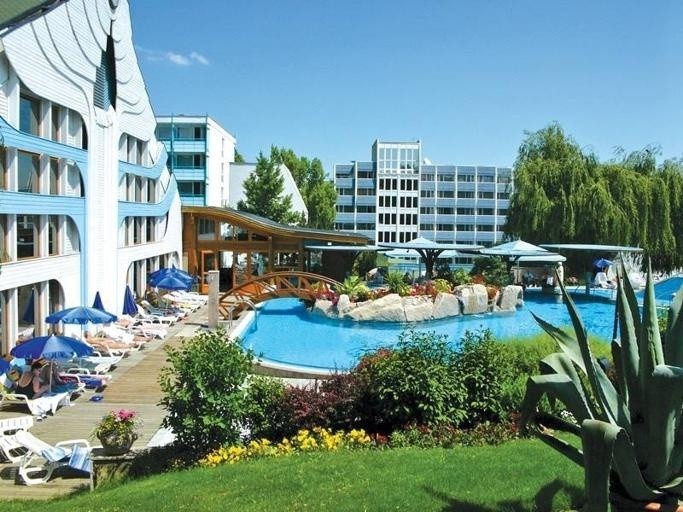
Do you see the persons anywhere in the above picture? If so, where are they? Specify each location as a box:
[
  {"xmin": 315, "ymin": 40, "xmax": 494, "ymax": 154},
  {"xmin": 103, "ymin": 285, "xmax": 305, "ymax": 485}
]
[
  {"xmin": 545, "ymin": 274, "xmax": 553, "ymax": 286},
  {"xmin": 84, "ymin": 331, "xmax": 139, "ymax": 349},
  {"xmin": 540, "ymin": 273, "xmax": 547, "ymax": 281},
  {"xmin": 16, "ymin": 362, "xmax": 49, "ymax": 401},
  {"xmin": 404, "ymin": 273, "xmax": 411, "ymax": 283},
  {"xmin": 42, "ymin": 361, "xmax": 63, "ymax": 385},
  {"xmin": 571, "ymin": 277, "xmax": 578, "ymax": 284},
  {"xmin": 116, "ymin": 319, "xmax": 144, "ymax": 330},
  {"xmin": 567, "ymin": 277, "xmax": 572, "ymax": 283},
  {"xmin": 525, "ymin": 271, "xmax": 533, "ymax": 281}
]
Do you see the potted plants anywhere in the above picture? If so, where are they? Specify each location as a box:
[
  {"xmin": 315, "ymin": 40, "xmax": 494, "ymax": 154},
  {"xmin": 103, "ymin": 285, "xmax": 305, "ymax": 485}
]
[{"xmin": 524, "ymin": 252, "xmax": 683, "ymax": 512}]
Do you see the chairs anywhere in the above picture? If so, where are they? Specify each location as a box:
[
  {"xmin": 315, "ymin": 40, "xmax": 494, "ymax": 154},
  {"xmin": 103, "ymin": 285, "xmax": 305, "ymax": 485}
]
[{"xmin": 1, "ymin": 291, "xmax": 208, "ymax": 485}]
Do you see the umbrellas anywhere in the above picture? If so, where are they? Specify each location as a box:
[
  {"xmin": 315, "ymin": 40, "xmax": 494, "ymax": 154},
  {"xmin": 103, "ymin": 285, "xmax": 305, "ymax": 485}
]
[
  {"xmin": 11, "ymin": 334, "xmax": 93, "ymax": 391},
  {"xmin": 92, "ymin": 292, "xmax": 104, "ymax": 307},
  {"xmin": 150, "ymin": 268, "xmax": 193, "ymax": 286},
  {"xmin": 23, "ymin": 289, "xmax": 35, "ymax": 326},
  {"xmin": 46, "ymin": 306, "xmax": 116, "ymax": 340},
  {"xmin": 123, "ymin": 285, "xmax": 138, "ymax": 316},
  {"xmin": 149, "ymin": 274, "xmax": 189, "ymax": 313},
  {"xmin": 593, "ymin": 258, "xmax": 614, "ymax": 272}
]
